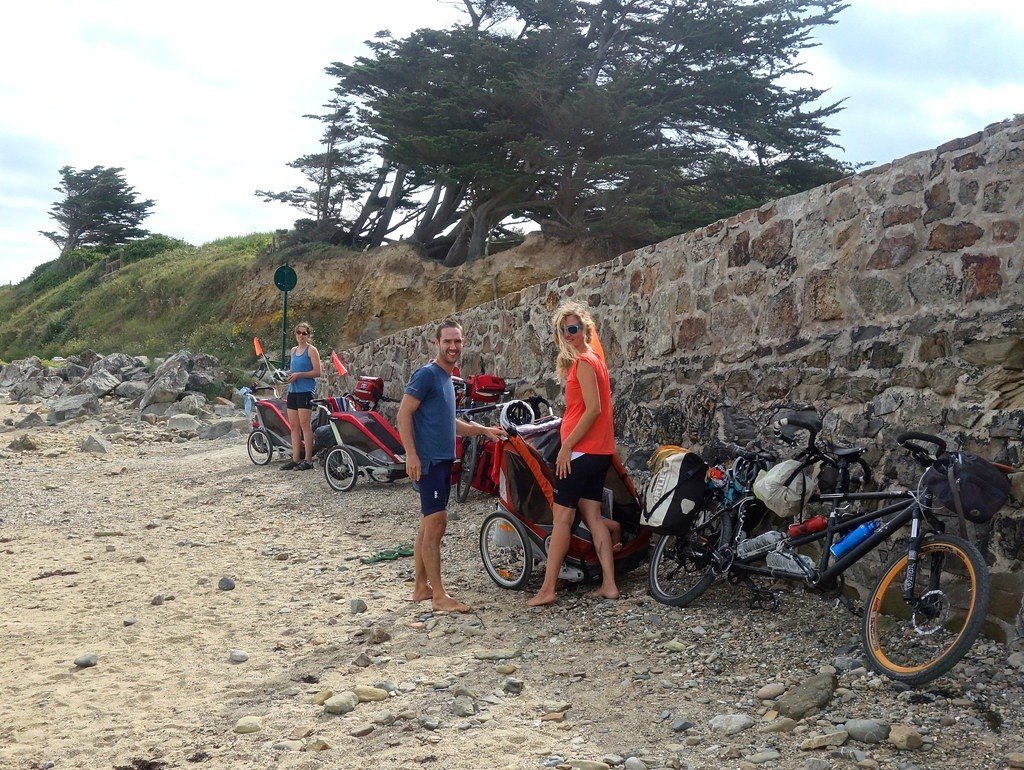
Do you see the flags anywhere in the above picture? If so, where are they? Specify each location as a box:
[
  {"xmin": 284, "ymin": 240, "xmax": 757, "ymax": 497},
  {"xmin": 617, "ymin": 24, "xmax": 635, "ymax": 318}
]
[
  {"xmin": 253, "ymin": 336, "xmax": 262, "ymax": 356},
  {"xmin": 332, "ymin": 351, "xmax": 347, "ymax": 376}
]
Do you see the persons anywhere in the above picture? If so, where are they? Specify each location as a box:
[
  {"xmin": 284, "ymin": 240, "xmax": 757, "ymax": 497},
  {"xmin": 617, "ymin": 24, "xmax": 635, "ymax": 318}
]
[
  {"xmin": 279, "ymin": 323, "xmax": 321, "ymax": 470},
  {"xmin": 396, "ymin": 320, "xmax": 507, "ymax": 613},
  {"xmin": 526, "ymin": 303, "xmax": 620, "ymax": 607}
]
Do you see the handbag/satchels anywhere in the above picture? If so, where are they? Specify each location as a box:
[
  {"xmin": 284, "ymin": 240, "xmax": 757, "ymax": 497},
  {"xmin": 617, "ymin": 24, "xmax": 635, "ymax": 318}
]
[
  {"xmin": 639, "ymin": 447, "xmax": 708, "ymax": 535},
  {"xmin": 924, "ymin": 449, "xmax": 1012, "ymax": 524},
  {"xmin": 756, "ymin": 458, "xmax": 817, "ymax": 518}
]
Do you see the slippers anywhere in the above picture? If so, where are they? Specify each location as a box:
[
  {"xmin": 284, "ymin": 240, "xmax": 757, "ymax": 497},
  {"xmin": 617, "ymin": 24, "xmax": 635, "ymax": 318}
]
[{"xmin": 360, "ymin": 540, "xmax": 413, "ymax": 564}]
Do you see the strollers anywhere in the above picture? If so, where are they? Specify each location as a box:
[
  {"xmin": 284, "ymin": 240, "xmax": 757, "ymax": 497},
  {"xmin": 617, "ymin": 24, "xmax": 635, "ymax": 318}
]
[
  {"xmin": 461, "ymin": 394, "xmax": 653, "ymax": 592},
  {"xmin": 309, "ymin": 394, "xmax": 407, "ymax": 491},
  {"xmin": 243, "ymin": 386, "xmax": 318, "ymax": 466}
]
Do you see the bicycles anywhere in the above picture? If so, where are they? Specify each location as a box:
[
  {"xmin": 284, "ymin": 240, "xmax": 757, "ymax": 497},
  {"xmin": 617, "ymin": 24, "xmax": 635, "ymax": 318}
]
[
  {"xmin": 648, "ymin": 408, "xmax": 1017, "ymax": 686},
  {"xmin": 451, "ymin": 375, "xmax": 507, "ymax": 503}
]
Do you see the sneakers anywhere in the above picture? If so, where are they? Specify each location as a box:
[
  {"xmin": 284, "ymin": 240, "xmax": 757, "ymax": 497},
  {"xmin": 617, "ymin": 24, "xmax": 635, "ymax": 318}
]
[
  {"xmin": 279, "ymin": 460, "xmax": 301, "ymax": 470},
  {"xmin": 293, "ymin": 460, "xmax": 314, "ymax": 471}
]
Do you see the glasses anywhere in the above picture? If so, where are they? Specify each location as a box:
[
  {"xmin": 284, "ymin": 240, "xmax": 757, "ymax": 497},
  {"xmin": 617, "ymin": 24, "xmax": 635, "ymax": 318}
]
[
  {"xmin": 560, "ymin": 324, "xmax": 585, "ymax": 334},
  {"xmin": 296, "ymin": 330, "xmax": 309, "ymax": 336}
]
